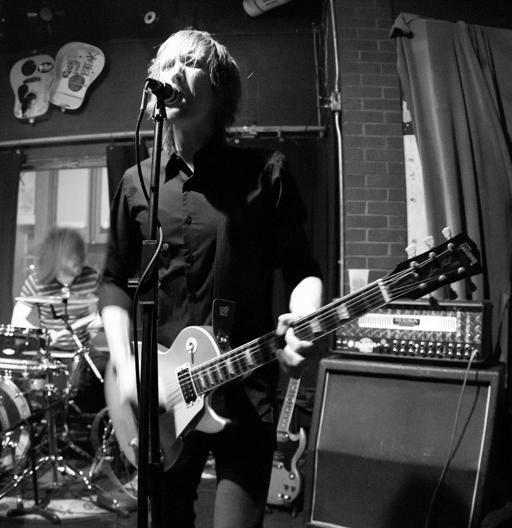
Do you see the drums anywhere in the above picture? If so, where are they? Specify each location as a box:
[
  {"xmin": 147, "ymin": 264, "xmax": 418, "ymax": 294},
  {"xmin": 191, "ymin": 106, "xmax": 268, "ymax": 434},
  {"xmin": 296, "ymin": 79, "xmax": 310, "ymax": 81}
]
[
  {"xmin": 1, "ymin": 377, "xmax": 33, "ymax": 475},
  {"xmin": 0, "ymin": 322, "xmax": 50, "ymax": 374}
]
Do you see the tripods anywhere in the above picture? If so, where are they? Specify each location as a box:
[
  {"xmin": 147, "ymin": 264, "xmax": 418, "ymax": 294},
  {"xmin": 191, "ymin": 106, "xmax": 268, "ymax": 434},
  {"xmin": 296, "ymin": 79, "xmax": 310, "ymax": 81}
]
[{"xmin": 0, "ymin": 373, "xmax": 133, "ymax": 528}]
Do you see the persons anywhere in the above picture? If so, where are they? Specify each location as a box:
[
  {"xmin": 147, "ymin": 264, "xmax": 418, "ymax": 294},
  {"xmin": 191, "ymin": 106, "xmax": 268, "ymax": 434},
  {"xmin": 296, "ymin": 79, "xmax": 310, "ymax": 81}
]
[
  {"xmin": 95, "ymin": 26, "xmax": 322, "ymax": 527},
  {"xmin": 8, "ymin": 229, "xmax": 106, "ymax": 423}
]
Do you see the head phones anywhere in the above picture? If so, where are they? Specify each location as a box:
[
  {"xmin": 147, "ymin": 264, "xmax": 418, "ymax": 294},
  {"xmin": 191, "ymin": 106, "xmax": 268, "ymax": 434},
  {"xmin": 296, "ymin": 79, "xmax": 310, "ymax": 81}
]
[{"xmin": 210, "ymin": 39, "xmax": 230, "ymax": 89}]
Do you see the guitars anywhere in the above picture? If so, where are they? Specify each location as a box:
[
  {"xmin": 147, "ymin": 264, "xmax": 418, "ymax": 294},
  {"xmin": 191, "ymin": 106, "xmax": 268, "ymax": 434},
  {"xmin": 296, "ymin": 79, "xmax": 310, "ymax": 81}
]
[
  {"xmin": 104, "ymin": 228, "xmax": 479, "ymax": 473},
  {"xmin": 265, "ymin": 376, "xmax": 308, "ymax": 510}
]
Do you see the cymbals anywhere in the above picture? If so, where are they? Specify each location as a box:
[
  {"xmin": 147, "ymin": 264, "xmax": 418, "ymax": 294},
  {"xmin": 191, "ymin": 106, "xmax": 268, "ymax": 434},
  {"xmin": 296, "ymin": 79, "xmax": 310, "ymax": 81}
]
[
  {"xmin": 13, "ymin": 295, "xmax": 99, "ymax": 306},
  {"xmin": 54, "ymin": 314, "xmax": 97, "ymax": 340}
]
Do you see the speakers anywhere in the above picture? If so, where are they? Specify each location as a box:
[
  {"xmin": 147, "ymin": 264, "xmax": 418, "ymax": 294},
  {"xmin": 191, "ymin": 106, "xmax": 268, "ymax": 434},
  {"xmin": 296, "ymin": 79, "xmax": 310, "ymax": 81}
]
[{"xmin": 302, "ymin": 353, "xmax": 505, "ymax": 528}]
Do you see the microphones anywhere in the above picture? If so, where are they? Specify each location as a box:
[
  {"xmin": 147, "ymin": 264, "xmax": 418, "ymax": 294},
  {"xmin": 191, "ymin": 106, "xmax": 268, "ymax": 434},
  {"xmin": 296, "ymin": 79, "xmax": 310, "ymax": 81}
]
[{"xmin": 144, "ymin": 78, "xmax": 182, "ymax": 108}]
